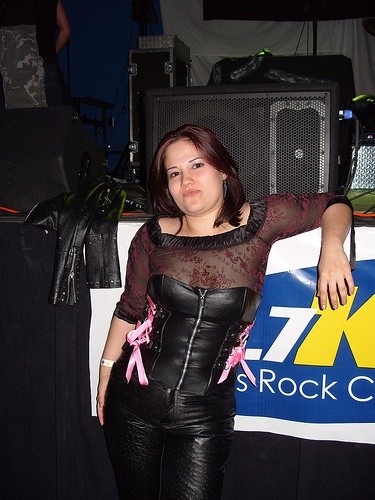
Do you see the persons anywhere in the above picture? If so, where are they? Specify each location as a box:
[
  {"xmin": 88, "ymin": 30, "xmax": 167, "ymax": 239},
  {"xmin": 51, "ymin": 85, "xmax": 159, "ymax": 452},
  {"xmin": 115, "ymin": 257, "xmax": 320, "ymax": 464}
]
[
  {"xmin": 98, "ymin": 124, "xmax": 355, "ymax": 500},
  {"xmin": 38, "ymin": 0, "xmax": 141, "ymax": 187}
]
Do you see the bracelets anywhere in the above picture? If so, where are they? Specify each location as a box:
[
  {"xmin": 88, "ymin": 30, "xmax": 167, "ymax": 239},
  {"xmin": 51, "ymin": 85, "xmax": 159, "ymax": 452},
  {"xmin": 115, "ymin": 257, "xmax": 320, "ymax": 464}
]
[{"xmin": 99, "ymin": 359, "xmax": 116, "ymax": 367}]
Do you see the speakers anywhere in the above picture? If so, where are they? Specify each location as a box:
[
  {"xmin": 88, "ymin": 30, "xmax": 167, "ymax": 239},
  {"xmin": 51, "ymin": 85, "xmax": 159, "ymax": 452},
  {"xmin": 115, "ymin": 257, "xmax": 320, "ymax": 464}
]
[{"xmin": 145, "ymin": 81, "xmax": 341, "ymax": 217}]
